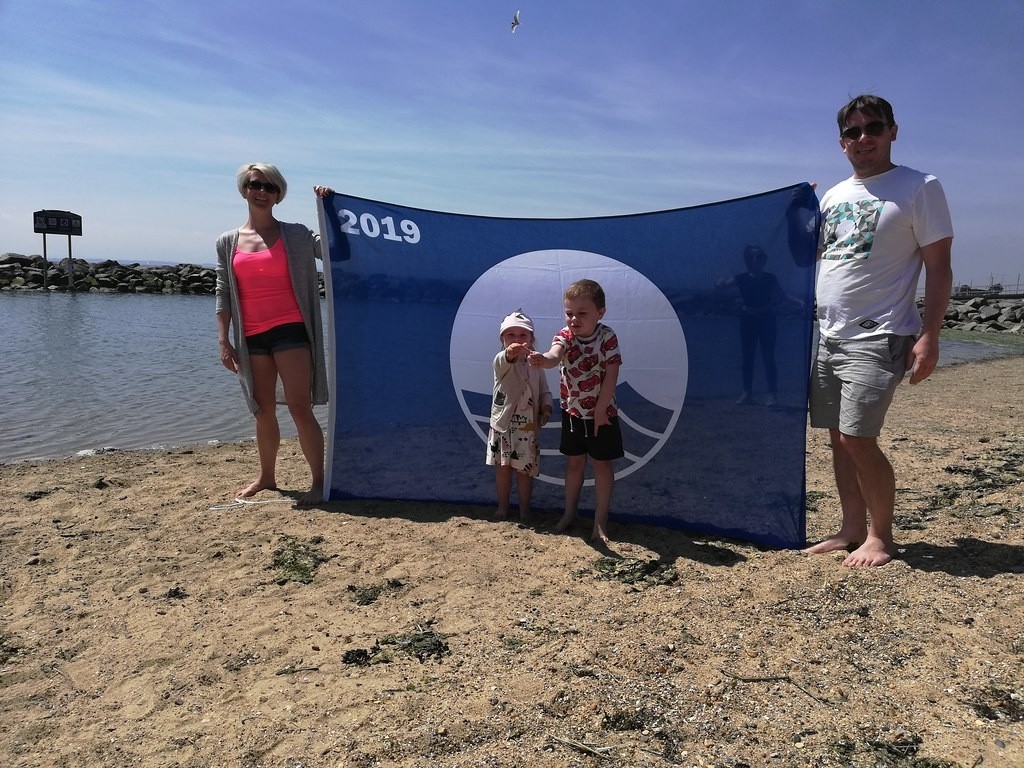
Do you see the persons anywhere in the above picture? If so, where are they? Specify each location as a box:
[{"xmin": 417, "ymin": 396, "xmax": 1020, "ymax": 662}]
[
  {"xmin": 786, "ymin": 95, "xmax": 954, "ymax": 566},
  {"xmin": 215, "ymin": 163, "xmax": 351, "ymax": 507},
  {"xmin": 520, "ymin": 279, "xmax": 624, "ymax": 544},
  {"xmin": 736, "ymin": 246, "xmax": 784, "ymax": 405},
  {"xmin": 486, "ymin": 308, "xmax": 553, "ymax": 522}
]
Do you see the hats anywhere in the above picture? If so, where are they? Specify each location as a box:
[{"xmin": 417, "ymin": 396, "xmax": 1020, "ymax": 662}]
[{"xmin": 499, "ymin": 310, "xmax": 534, "ymax": 334}]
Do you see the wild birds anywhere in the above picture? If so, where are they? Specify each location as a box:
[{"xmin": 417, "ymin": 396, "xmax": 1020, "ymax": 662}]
[{"xmin": 511, "ymin": 8, "xmax": 523, "ymax": 34}]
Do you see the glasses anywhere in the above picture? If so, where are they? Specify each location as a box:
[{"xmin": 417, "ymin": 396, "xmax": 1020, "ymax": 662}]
[
  {"xmin": 246, "ymin": 180, "xmax": 278, "ymax": 193},
  {"xmin": 842, "ymin": 120, "xmax": 891, "ymax": 140}
]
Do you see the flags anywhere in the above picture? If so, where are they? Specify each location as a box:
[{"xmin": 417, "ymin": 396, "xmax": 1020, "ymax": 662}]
[{"xmin": 317, "ymin": 182, "xmax": 821, "ymax": 548}]
